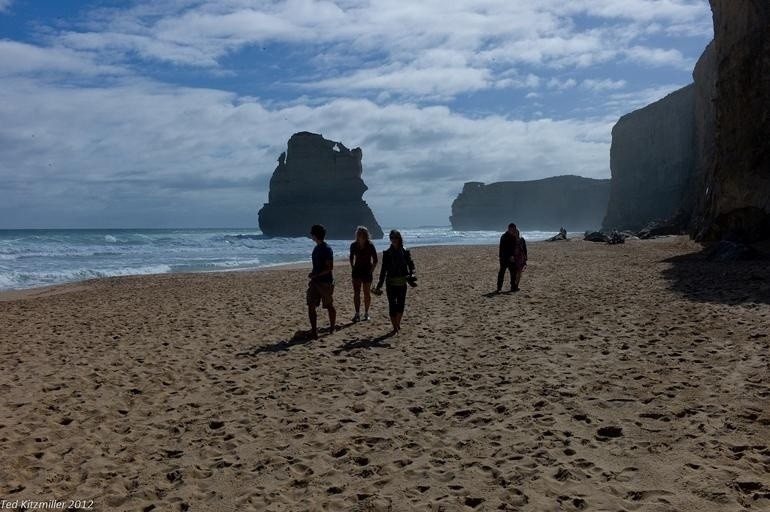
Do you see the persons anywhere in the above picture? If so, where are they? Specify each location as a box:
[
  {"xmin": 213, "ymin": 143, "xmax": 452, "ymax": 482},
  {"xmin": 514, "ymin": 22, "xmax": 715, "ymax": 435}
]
[
  {"xmin": 377, "ymin": 229, "xmax": 417, "ymax": 335},
  {"xmin": 497, "ymin": 221, "xmax": 522, "ymax": 295},
  {"xmin": 350, "ymin": 224, "xmax": 378, "ymax": 322},
  {"xmin": 513, "ymin": 229, "xmax": 528, "ymax": 286},
  {"xmin": 303, "ymin": 223, "xmax": 339, "ymax": 342}
]
